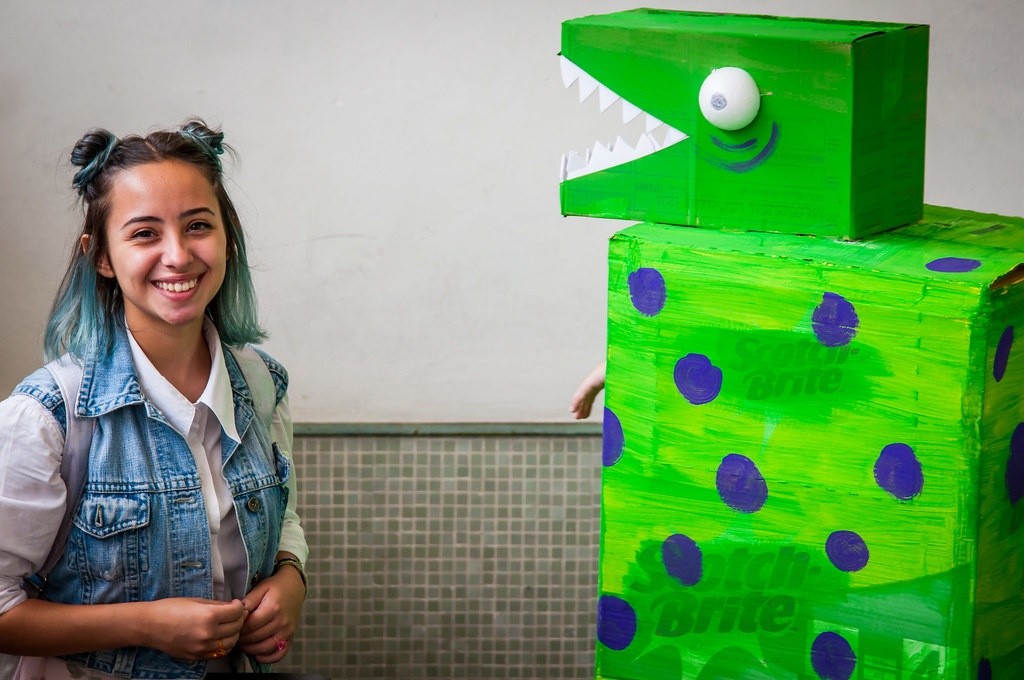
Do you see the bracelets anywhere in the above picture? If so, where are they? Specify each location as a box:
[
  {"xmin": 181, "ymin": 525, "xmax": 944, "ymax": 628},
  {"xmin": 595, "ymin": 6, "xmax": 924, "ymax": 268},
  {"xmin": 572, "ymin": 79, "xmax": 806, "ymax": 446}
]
[{"xmin": 277, "ymin": 558, "xmax": 309, "ymax": 609}]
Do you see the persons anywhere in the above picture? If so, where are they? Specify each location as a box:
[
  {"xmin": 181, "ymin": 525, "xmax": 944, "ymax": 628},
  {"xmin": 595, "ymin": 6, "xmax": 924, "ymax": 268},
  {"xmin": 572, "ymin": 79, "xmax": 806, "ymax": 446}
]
[
  {"xmin": 569, "ymin": 360, "xmax": 607, "ymax": 419},
  {"xmin": 0, "ymin": 116, "xmax": 310, "ymax": 680}
]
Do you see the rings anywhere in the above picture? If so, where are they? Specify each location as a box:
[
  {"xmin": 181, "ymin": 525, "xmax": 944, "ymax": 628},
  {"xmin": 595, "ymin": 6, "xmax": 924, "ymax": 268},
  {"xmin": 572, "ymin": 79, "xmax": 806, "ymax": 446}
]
[
  {"xmin": 212, "ymin": 639, "xmax": 225, "ymax": 658},
  {"xmin": 271, "ymin": 635, "xmax": 287, "ymax": 650}
]
